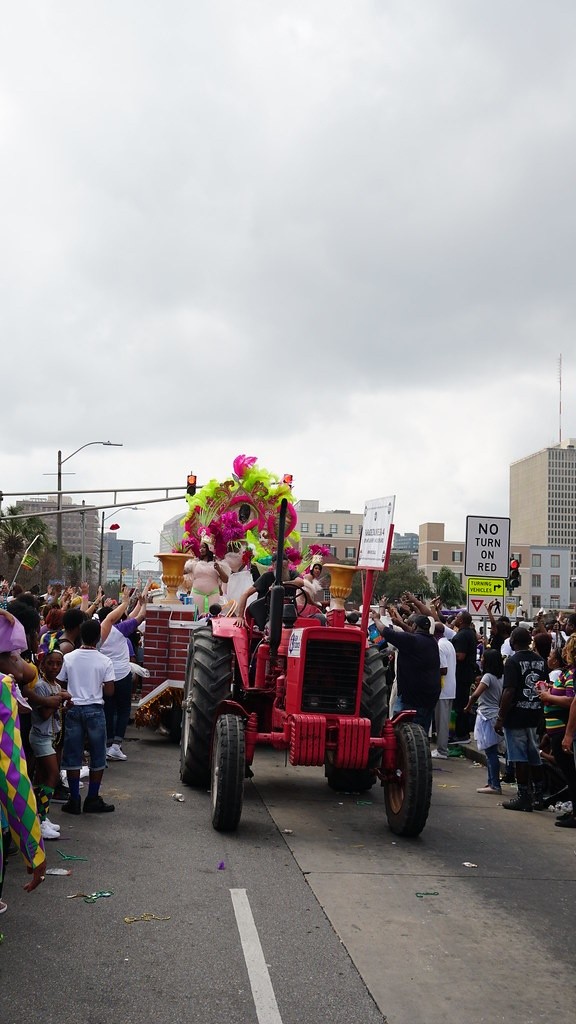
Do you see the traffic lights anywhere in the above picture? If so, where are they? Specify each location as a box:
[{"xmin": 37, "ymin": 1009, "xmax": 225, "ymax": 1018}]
[
  {"xmin": 187, "ymin": 476, "xmax": 196, "ymax": 501},
  {"xmin": 507, "ymin": 558, "xmax": 520, "ymax": 588},
  {"xmin": 283, "ymin": 473, "xmax": 293, "ymax": 491}
]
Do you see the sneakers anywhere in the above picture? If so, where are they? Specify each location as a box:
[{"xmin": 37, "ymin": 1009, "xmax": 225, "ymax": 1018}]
[
  {"xmin": 61, "ymin": 796, "xmax": 81, "ymax": 814},
  {"xmin": 39, "ymin": 817, "xmax": 60, "ymax": 838},
  {"xmin": 106, "ymin": 748, "xmax": 128, "ymax": 761},
  {"xmin": 84, "ymin": 796, "xmax": 115, "ymax": 812},
  {"xmin": 49, "ymin": 776, "xmax": 69, "ymax": 803},
  {"xmin": 503, "ymin": 794, "xmax": 534, "ymax": 812},
  {"xmin": 533, "ymin": 799, "xmax": 544, "ymax": 811}
]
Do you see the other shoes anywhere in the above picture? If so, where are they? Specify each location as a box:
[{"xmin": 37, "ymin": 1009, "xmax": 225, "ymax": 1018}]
[
  {"xmin": 556, "ymin": 812, "xmax": 576, "ymax": 820},
  {"xmin": 448, "ymin": 734, "xmax": 472, "ymax": 744},
  {"xmin": 0, "ymin": 898, "xmax": 7, "ymax": 913},
  {"xmin": 431, "ymin": 748, "xmax": 447, "ymax": 760},
  {"xmin": 555, "ymin": 817, "xmax": 576, "ymax": 827},
  {"xmin": 8, "ymin": 847, "xmax": 19, "ymax": 855},
  {"xmin": 62, "ymin": 778, "xmax": 84, "ymax": 789},
  {"xmin": 477, "ymin": 786, "xmax": 502, "ymax": 795}
]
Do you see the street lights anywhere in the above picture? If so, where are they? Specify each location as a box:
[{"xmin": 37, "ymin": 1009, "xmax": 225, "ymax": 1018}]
[
  {"xmin": 97, "ymin": 506, "xmax": 154, "ymax": 602},
  {"xmin": 56, "ymin": 440, "xmax": 123, "ymax": 582}
]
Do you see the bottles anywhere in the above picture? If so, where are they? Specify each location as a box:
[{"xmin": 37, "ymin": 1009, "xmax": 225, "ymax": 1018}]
[{"xmin": 172, "ymin": 794, "xmax": 184, "ymax": 801}]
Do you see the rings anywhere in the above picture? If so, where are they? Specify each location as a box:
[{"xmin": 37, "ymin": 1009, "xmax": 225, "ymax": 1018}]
[{"xmin": 565, "ymin": 749, "xmax": 567, "ymax": 751}]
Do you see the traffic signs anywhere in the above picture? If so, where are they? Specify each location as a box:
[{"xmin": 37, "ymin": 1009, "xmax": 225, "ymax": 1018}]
[{"xmin": 467, "ymin": 577, "xmax": 505, "ymax": 596}]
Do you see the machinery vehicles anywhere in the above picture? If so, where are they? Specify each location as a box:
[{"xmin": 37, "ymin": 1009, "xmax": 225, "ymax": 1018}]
[{"xmin": 178, "ymin": 498, "xmax": 433, "ymax": 837}]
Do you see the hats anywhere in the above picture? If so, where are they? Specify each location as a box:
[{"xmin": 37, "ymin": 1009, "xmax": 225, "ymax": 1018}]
[{"xmin": 410, "ymin": 615, "xmax": 431, "ymax": 630}]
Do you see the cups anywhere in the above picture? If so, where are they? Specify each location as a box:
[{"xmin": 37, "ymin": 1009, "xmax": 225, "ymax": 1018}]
[{"xmin": 536, "ymin": 682, "xmax": 551, "ymax": 706}]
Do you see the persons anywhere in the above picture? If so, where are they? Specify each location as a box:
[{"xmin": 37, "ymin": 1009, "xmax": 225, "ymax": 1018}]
[
  {"xmin": 232, "ymin": 554, "xmax": 305, "ymax": 648},
  {"xmin": 294, "ymin": 562, "xmax": 325, "ymax": 617},
  {"xmin": 187, "ymin": 539, "xmax": 229, "ymax": 614},
  {"xmin": 351, "ymin": 589, "xmax": 576, "ymax": 828},
  {"xmin": 0, "ymin": 574, "xmax": 153, "ymax": 914},
  {"xmin": 222, "ymin": 541, "xmax": 248, "ymax": 594}
]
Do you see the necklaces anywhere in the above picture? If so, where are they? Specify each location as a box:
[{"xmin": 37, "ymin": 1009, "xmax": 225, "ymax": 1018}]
[{"xmin": 43, "ymin": 674, "xmax": 63, "ymax": 748}]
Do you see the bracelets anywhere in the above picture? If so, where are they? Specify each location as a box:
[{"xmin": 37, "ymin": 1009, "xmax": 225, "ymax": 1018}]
[
  {"xmin": 92, "ymin": 602, "xmax": 98, "ymax": 607},
  {"xmin": 380, "ymin": 605, "xmax": 384, "ymax": 607},
  {"xmin": 539, "ymin": 750, "xmax": 543, "ymax": 753},
  {"xmin": 82, "ymin": 594, "xmax": 88, "ymax": 599},
  {"xmin": 496, "ymin": 714, "xmax": 502, "ymax": 720}
]
